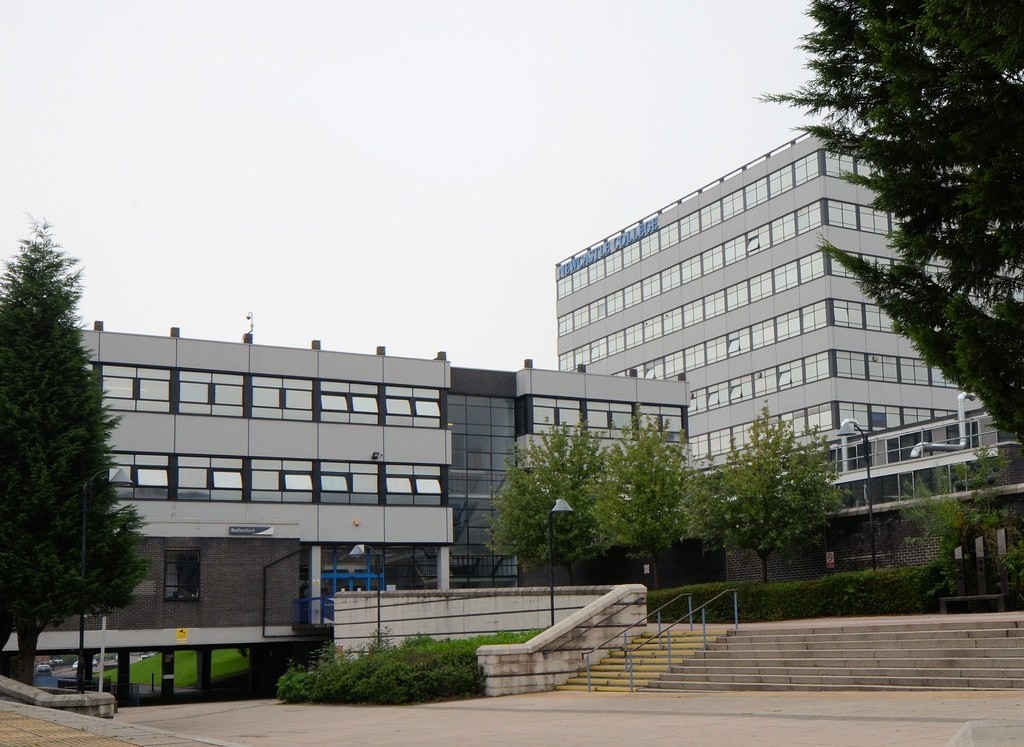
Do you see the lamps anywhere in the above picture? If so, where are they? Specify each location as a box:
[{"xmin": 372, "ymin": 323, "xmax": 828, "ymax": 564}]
[{"xmin": 372, "ymin": 452, "xmax": 379, "ymax": 459}]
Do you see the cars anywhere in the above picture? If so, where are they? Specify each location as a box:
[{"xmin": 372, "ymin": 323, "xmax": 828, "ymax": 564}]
[
  {"xmin": 71, "ymin": 661, "xmax": 79, "ymax": 671},
  {"xmin": 35, "ymin": 665, "xmax": 51, "ymax": 676},
  {"xmin": 92, "ymin": 659, "xmax": 98, "ymax": 667}
]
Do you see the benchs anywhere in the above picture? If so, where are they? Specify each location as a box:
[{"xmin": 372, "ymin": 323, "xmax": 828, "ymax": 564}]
[{"xmin": 940, "ymin": 594, "xmax": 1004, "ymax": 613}]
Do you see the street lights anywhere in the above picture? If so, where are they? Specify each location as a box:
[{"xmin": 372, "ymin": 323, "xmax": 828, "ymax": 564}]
[
  {"xmin": 348, "ymin": 544, "xmax": 381, "ymax": 646},
  {"xmin": 835, "ymin": 420, "xmax": 878, "ymax": 572},
  {"xmin": 76, "ymin": 466, "xmax": 134, "ymax": 695},
  {"xmin": 550, "ymin": 498, "xmax": 573, "ymax": 627}
]
